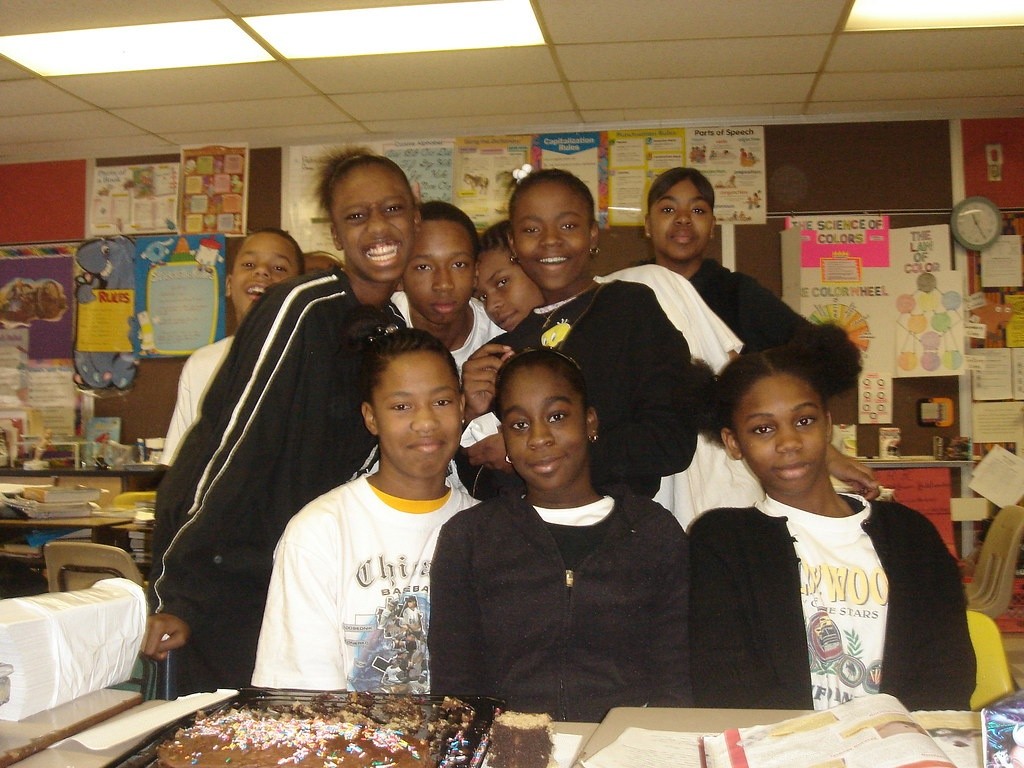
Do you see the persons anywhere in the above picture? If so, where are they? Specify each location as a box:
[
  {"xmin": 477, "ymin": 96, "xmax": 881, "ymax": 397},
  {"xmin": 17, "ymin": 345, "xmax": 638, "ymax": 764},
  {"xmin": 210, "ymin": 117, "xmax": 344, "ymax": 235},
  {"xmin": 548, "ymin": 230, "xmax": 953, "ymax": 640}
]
[
  {"xmin": 671, "ymin": 318, "xmax": 979, "ymax": 722},
  {"xmin": 136, "ymin": 146, "xmax": 421, "ymax": 701},
  {"xmin": 634, "ymin": 165, "xmax": 816, "ymax": 356},
  {"xmin": 428, "ymin": 345, "xmax": 695, "ymax": 724},
  {"xmin": 249, "ymin": 324, "xmax": 491, "ymax": 696},
  {"xmin": 471, "ymin": 217, "xmax": 885, "ymax": 537},
  {"xmin": 157, "ymin": 225, "xmax": 305, "ymax": 485},
  {"xmin": 301, "ymin": 249, "xmax": 344, "ymax": 275},
  {"xmin": 383, "ymin": 199, "xmax": 507, "ymax": 388},
  {"xmin": 451, "ymin": 169, "xmax": 705, "ymax": 507}
]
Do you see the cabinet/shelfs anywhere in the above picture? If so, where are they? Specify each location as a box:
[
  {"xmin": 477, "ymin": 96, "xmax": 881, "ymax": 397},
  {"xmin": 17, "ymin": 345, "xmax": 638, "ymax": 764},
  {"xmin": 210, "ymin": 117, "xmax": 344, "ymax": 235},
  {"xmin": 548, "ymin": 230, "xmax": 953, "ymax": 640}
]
[{"xmin": 0, "ymin": 462, "xmax": 165, "ymax": 496}]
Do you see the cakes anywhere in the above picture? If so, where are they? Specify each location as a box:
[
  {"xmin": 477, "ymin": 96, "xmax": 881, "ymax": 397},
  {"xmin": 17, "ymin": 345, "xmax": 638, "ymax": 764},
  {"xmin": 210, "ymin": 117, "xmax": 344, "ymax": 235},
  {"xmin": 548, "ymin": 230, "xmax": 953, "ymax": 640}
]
[{"xmin": 155, "ymin": 713, "xmax": 429, "ymax": 768}]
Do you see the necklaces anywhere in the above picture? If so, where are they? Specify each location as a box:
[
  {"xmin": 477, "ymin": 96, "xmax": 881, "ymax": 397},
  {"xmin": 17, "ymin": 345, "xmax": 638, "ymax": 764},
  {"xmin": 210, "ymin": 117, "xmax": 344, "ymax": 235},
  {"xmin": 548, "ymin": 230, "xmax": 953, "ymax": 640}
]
[{"xmin": 538, "ymin": 278, "xmax": 595, "ymax": 329}]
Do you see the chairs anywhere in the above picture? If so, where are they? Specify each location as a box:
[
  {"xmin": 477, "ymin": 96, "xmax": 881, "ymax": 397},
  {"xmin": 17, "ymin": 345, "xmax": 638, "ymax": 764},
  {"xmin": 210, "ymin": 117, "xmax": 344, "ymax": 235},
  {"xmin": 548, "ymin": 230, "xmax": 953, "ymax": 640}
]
[
  {"xmin": 43, "ymin": 541, "xmax": 146, "ymax": 593},
  {"xmin": 965, "ymin": 610, "xmax": 1015, "ymax": 713},
  {"xmin": 963, "ymin": 505, "xmax": 1024, "ymax": 621}
]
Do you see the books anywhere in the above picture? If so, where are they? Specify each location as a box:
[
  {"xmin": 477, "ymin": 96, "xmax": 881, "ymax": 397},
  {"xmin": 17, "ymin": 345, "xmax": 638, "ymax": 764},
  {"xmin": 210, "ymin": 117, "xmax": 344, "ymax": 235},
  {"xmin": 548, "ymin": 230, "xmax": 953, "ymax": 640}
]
[
  {"xmin": 696, "ymin": 690, "xmax": 981, "ymax": 768},
  {"xmin": 1, "ymin": 479, "xmax": 154, "ymax": 564}
]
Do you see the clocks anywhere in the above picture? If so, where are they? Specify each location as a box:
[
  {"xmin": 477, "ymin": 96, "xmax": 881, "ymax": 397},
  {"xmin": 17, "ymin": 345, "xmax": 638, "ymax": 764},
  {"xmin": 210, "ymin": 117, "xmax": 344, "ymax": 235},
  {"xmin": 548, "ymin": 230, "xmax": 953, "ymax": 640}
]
[{"xmin": 950, "ymin": 196, "xmax": 1003, "ymax": 251}]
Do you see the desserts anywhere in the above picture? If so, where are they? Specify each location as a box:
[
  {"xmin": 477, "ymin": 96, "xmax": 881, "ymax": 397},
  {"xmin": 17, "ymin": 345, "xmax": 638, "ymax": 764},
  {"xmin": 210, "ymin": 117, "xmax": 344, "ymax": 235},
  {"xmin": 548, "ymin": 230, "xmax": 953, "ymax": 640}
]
[{"xmin": 487, "ymin": 711, "xmax": 557, "ymax": 768}]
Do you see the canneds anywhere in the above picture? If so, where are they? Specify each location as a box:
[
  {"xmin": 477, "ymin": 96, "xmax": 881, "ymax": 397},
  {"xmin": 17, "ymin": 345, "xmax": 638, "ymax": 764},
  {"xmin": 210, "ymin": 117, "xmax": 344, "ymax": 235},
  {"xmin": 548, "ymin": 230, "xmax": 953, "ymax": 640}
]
[{"xmin": 879, "ymin": 428, "xmax": 900, "ymax": 459}]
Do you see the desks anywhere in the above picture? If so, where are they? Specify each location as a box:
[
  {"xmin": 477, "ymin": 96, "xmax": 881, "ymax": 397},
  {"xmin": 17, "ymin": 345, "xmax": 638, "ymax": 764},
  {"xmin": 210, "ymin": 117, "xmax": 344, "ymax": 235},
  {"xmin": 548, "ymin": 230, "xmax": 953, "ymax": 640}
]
[
  {"xmin": 110, "ymin": 522, "xmax": 155, "ymax": 566},
  {"xmin": 0, "ymin": 515, "xmax": 134, "ymax": 564},
  {"xmin": 1, "ymin": 687, "xmax": 143, "ymax": 768},
  {"xmin": 573, "ymin": 707, "xmax": 823, "ymax": 768},
  {"xmin": 5, "ymin": 700, "xmax": 602, "ymax": 768}
]
